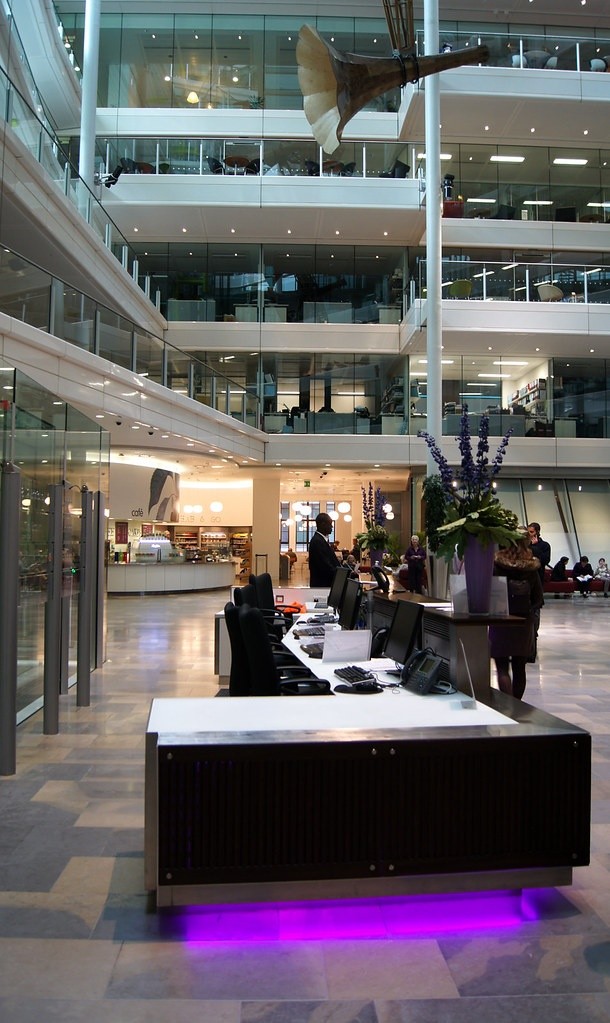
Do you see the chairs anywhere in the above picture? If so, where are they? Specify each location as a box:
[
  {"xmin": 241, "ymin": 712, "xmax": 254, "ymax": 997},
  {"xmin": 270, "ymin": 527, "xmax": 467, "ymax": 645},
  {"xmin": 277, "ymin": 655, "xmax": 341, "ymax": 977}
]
[
  {"xmin": 248, "ymin": 572, "xmax": 301, "ymax": 619},
  {"xmin": 555, "ymin": 205, "xmax": 577, "ymax": 222},
  {"xmin": 488, "ymin": 203, "xmax": 518, "ymax": 219},
  {"xmin": 236, "ymin": 604, "xmax": 332, "ymax": 696},
  {"xmin": 225, "ymin": 601, "xmax": 239, "ymax": 697},
  {"xmin": 232, "ymin": 585, "xmax": 285, "ymax": 642},
  {"xmin": 508, "ymin": 52, "xmax": 608, "ymax": 72},
  {"xmin": 538, "ymin": 284, "xmax": 564, "ymax": 302},
  {"xmin": 121, "ymin": 157, "xmax": 411, "ymax": 178},
  {"xmin": 449, "ymin": 280, "xmax": 479, "ymax": 297}
]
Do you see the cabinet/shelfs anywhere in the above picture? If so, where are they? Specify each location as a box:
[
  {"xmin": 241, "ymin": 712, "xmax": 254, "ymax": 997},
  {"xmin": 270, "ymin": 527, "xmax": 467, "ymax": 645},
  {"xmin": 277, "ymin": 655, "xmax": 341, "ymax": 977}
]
[{"xmin": 155, "ymin": 526, "xmax": 253, "ymax": 579}]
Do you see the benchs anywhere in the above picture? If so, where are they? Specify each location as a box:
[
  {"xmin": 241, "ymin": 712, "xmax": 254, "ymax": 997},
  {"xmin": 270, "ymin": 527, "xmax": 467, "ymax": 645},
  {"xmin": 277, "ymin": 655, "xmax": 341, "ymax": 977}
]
[{"xmin": 544, "ymin": 569, "xmax": 610, "ymax": 593}]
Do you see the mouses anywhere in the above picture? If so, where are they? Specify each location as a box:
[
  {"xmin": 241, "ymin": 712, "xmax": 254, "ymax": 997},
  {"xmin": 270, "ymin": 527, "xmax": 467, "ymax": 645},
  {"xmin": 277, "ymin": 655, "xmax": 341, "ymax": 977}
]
[
  {"xmin": 314, "ymin": 598, "xmax": 318, "ymax": 602},
  {"xmin": 356, "ymin": 683, "xmax": 377, "ymax": 691},
  {"xmin": 309, "ymin": 652, "xmax": 322, "ymax": 658},
  {"xmin": 310, "ymin": 619, "xmax": 320, "ymax": 623}
]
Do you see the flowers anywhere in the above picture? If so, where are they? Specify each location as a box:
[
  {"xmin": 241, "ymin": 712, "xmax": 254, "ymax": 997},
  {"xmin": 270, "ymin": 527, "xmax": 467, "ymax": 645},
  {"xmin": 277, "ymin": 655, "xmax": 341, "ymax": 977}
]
[
  {"xmin": 417, "ymin": 405, "xmax": 534, "ymax": 564},
  {"xmin": 353, "ymin": 481, "xmax": 401, "ymax": 558}
]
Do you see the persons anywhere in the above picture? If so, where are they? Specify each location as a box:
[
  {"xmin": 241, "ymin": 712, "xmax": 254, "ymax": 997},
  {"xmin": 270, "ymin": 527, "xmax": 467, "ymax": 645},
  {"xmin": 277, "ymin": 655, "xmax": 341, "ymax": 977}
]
[
  {"xmin": 405, "ymin": 535, "xmax": 427, "ymax": 593},
  {"xmin": 592, "ymin": 558, "xmax": 610, "ymax": 598},
  {"xmin": 163, "ymin": 529, "xmax": 170, "ymax": 539},
  {"xmin": 284, "ymin": 548, "xmax": 297, "ymax": 570},
  {"xmin": 331, "ymin": 537, "xmax": 408, "ymax": 583},
  {"xmin": 571, "ymin": 556, "xmax": 595, "ymax": 598},
  {"xmin": 488, "ymin": 522, "xmax": 551, "ymax": 699},
  {"xmin": 308, "ymin": 513, "xmax": 341, "ymax": 587},
  {"xmin": 550, "ymin": 556, "xmax": 574, "ymax": 599},
  {"xmin": 441, "ymin": 42, "xmax": 453, "ymax": 53}
]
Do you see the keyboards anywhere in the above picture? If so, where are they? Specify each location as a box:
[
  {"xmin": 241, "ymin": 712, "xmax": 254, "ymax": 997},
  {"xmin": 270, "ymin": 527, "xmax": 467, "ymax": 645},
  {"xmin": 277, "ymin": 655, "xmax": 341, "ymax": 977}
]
[{"xmin": 298, "ymin": 602, "xmax": 376, "ymax": 685}]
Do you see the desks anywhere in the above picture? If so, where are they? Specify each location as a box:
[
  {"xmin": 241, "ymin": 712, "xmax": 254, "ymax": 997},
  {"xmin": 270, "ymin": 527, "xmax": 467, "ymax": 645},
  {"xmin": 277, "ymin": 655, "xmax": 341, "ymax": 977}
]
[
  {"xmin": 131, "ymin": 160, "xmax": 157, "ymax": 175},
  {"xmin": 528, "ymin": 51, "xmax": 553, "ymax": 69},
  {"xmin": 317, "ymin": 158, "xmax": 345, "ymax": 178},
  {"xmin": 221, "ymin": 155, "xmax": 249, "ymax": 176}
]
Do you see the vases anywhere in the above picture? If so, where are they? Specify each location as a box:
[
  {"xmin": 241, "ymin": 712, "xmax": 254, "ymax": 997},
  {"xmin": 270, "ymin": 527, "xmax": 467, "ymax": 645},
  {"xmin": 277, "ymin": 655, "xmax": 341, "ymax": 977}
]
[
  {"xmin": 461, "ymin": 532, "xmax": 495, "ymax": 615},
  {"xmin": 368, "ymin": 547, "xmax": 385, "ymax": 579}
]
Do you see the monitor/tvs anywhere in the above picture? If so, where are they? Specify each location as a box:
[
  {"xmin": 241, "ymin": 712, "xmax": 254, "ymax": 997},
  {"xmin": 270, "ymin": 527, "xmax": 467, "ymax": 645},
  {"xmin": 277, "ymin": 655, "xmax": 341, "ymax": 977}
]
[{"xmin": 328, "ymin": 567, "xmax": 424, "ymax": 678}]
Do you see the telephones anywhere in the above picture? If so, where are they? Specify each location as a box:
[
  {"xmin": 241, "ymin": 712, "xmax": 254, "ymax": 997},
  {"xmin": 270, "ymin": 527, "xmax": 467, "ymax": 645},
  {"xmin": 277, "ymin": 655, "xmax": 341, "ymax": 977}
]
[{"xmin": 399, "ymin": 651, "xmax": 443, "ymax": 696}]
